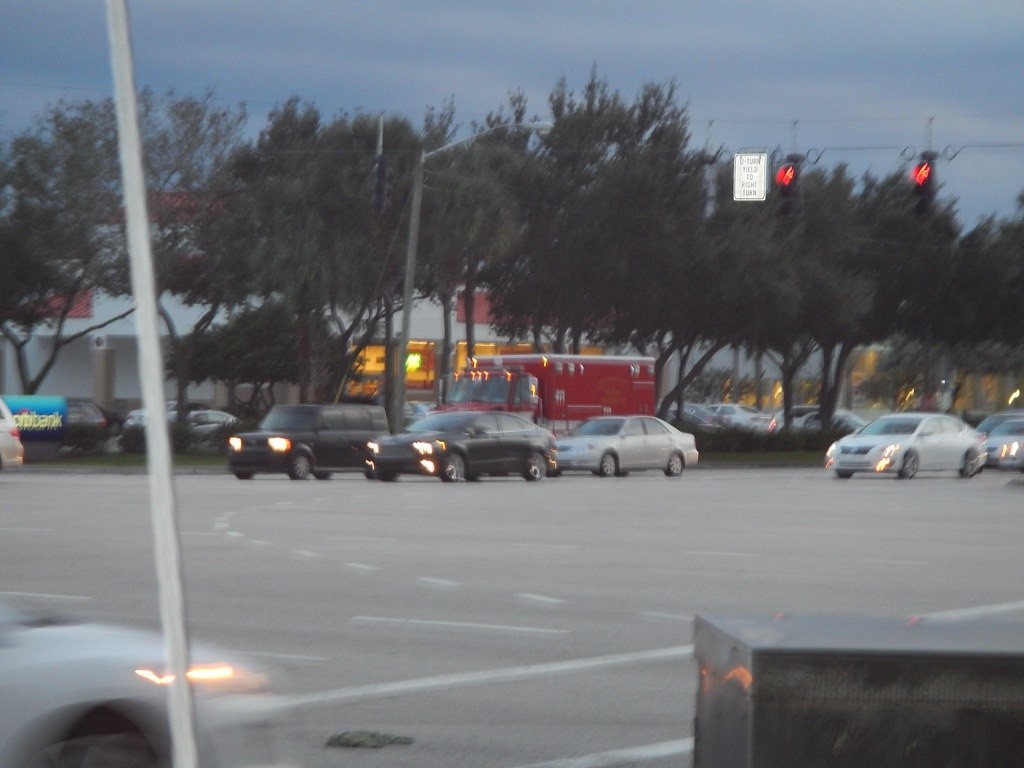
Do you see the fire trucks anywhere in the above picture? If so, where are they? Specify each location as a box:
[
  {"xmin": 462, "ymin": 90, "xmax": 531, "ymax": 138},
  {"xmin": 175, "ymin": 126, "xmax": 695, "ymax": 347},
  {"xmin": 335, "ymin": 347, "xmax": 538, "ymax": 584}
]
[{"xmin": 437, "ymin": 352, "xmax": 657, "ymax": 442}]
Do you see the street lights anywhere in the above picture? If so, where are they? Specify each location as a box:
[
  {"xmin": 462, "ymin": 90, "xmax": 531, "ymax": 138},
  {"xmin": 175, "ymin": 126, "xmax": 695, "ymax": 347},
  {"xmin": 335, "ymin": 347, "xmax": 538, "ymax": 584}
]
[{"xmin": 393, "ymin": 116, "xmax": 552, "ymax": 430}]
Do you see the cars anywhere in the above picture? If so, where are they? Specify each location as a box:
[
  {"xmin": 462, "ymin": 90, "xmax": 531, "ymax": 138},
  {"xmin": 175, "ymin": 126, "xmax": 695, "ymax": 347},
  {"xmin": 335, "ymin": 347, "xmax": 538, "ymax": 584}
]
[
  {"xmin": 366, "ymin": 410, "xmax": 560, "ymax": 483},
  {"xmin": 708, "ymin": 404, "xmax": 772, "ymax": 434},
  {"xmin": 551, "ymin": 415, "xmax": 698, "ymax": 476},
  {"xmin": 1, "ymin": 620, "xmax": 273, "ymax": 768},
  {"xmin": 825, "ymin": 413, "xmax": 988, "ymax": 482},
  {"xmin": 986, "ymin": 420, "xmax": 1024, "ymax": 475},
  {"xmin": 769, "ymin": 405, "xmax": 848, "ymax": 435},
  {"xmin": 793, "ymin": 411, "xmax": 871, "ymax": 433},
  {"xmin": 976, "ymin": 409, "xmax": 1023, "ymax": 440},
  {"xmin": 125, "ymin": 399, "xmax": 231, "ymax": 446},
  {"xmin": 65, "ymin": 398, "xmax": 125, "ymax": 438},
  {"xmin": 658, "ymin": 400, "xmax": 733, "ymax": 436},
  {"xmin": 0, "ymin": 398, "xmax": 23, "ymax": 471}
]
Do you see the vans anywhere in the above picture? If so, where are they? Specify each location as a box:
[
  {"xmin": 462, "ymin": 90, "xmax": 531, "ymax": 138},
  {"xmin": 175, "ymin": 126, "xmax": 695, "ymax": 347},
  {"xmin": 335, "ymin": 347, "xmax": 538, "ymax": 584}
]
[{"xmin": 227, "ymin": 406, "xmax": 394, "ymax": 480}]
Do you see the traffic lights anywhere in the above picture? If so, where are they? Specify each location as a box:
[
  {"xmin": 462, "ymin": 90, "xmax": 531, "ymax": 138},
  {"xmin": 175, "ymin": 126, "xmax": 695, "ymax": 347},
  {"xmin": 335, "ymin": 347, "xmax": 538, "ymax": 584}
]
[
  {"xmin": 776, "ymin": 153, "xmax": 803, "ymax": 224},
  {"xmin": 910, "ymin": 151, "xmax": 936, "ymax": 219}
]
